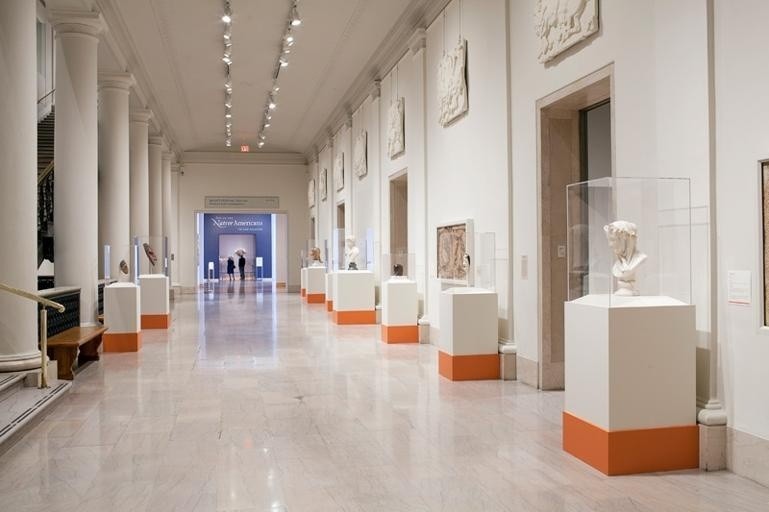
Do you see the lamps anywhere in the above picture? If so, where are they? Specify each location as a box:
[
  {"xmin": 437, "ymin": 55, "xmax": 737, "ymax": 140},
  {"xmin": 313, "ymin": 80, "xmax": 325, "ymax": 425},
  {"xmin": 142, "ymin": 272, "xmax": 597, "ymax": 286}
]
[{"xmin": 216, "ymin": 0, "xmax": 305, "ymax": 152}]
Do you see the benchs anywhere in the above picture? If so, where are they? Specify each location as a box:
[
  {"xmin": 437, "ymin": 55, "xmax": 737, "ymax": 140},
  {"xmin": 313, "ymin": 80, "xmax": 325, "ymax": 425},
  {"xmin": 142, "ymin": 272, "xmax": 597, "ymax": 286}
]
[{"xmin": 37, "ymin": 324, "xmax": 111, "ymax": 384}]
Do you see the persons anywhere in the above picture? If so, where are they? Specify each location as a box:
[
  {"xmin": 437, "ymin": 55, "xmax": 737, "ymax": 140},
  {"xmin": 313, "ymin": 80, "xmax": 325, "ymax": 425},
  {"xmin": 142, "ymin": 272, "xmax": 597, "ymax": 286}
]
[
  {"xmin": 604, "ymin": 221, "xmax": 647, "ymax": 280},
  {"xmin": 227, "ymin": 257, "xmax": 236, "ymax": 280},
  {"xmin": 238, "ymin": 255, "xmax": 245, "ymax": 279},
  {"xmin": 344, "ymin": 236, "xmax": 360, "ymax": 262},
  {"xmin": 462, "ymin": 253, "xmax": 470, "ymax": 270},
  {"xmin": 310, "ymin": 246, "xmax": 320, "ymax": 260}
]
